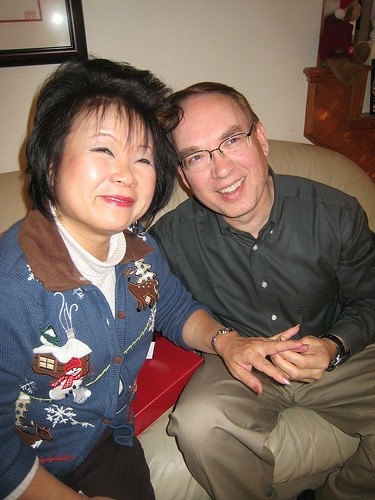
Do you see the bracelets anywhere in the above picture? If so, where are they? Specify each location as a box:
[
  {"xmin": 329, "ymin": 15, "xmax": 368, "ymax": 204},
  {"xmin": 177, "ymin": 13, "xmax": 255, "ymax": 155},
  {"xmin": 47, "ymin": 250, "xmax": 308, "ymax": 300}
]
[
  {"xmin": 78, "ymin": 490, "xmax": 85, "ymax": 500},
  {"xmin": 212, "ymin": 327, "xmax": 236, "ymax": 355}
]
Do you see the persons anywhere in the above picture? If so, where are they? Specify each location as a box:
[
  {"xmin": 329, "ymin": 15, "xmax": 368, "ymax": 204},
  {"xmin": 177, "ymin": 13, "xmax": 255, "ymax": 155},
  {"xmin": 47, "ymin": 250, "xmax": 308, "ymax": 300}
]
[
  {"xmin": 146, "ymin": 82, "xmax": 375, "ymax": 500},
  {"xmin": 0, "ymin": 57, "xmax": 309, "ymax": 500}
]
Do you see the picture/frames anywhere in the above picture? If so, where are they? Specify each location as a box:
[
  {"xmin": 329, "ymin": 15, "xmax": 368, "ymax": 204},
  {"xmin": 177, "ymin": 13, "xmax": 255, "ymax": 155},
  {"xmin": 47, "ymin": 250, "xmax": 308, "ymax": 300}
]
[{"xmin": 0, "ymin": 0, "xmax": 89, "ymax": 68}]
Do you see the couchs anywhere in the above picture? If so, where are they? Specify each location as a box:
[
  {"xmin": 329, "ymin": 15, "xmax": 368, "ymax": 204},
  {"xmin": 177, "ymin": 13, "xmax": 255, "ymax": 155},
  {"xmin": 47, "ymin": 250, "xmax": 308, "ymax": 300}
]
[{"xmin": 0, "ymin": 139, "xmax": 375, "ymax": 500}]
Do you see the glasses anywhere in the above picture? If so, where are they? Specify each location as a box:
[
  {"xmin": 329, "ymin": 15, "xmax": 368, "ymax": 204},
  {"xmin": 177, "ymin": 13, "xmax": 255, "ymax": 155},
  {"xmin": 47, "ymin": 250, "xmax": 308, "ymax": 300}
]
[{"xmin": 178, "ymin": 120, "xmax": 254, "ymax": 172}]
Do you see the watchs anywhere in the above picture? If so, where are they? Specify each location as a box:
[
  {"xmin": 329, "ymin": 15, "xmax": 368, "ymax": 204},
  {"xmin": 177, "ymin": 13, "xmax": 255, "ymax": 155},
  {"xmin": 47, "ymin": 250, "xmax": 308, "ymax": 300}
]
[{"xmin": 319, "ymin": 331, "xmax": 349, "ymax": 372}]
